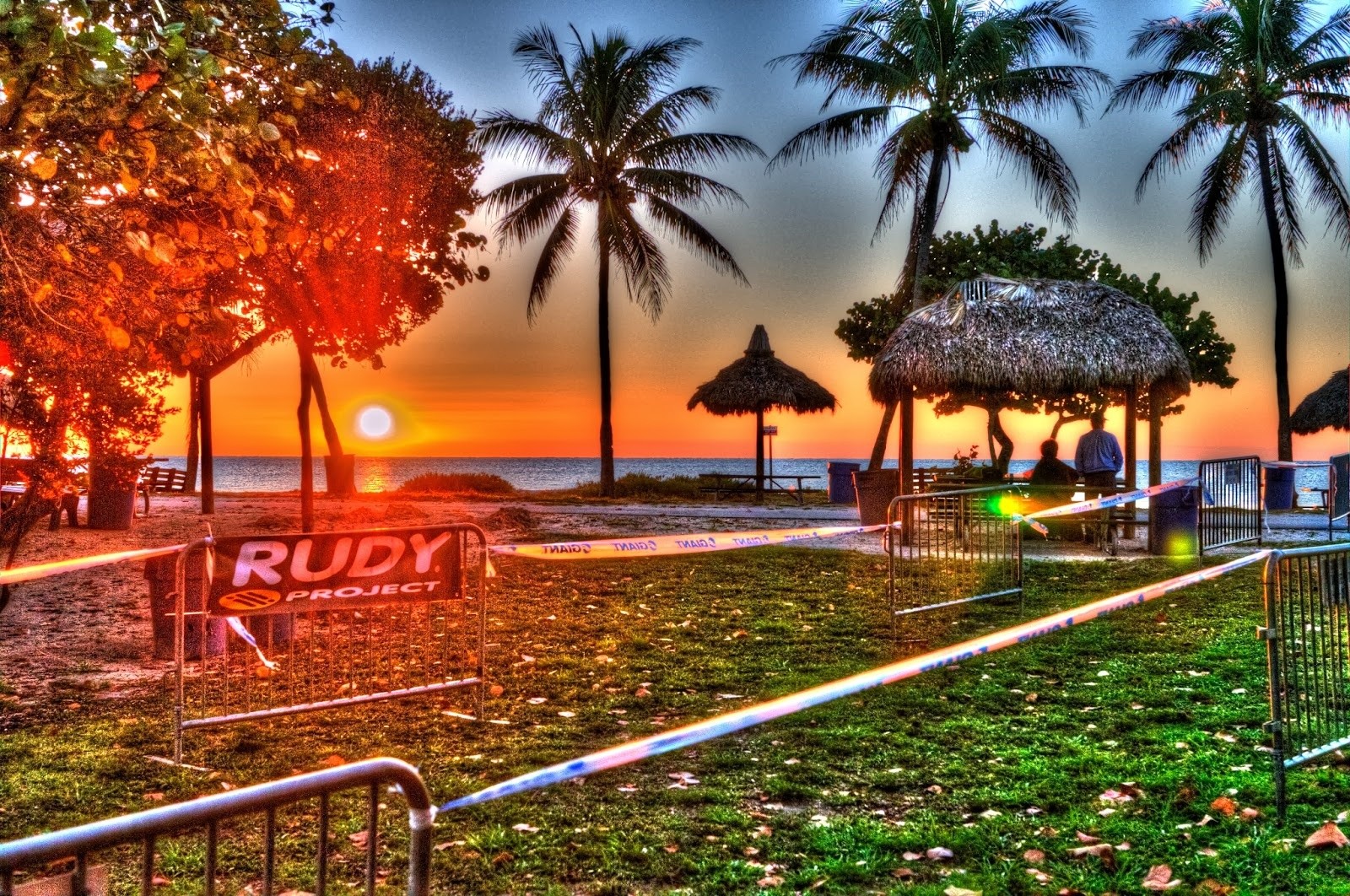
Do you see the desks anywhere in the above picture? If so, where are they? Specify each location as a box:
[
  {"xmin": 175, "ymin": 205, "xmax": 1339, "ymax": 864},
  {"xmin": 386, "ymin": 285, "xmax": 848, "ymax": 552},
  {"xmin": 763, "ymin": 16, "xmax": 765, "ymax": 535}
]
[{"xmin": 700, "ymin": 474, "xmax": 822, "ymax": 503}]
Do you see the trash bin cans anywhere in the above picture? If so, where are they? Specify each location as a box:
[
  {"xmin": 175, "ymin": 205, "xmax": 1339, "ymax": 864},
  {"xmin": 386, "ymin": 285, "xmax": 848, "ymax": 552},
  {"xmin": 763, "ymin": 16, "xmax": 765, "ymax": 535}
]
[
  {"xmin": 236, "ymin": 613, "xmax": 297, "ymax": 645},
  {"xmin": 827, "ymin": 462, "xmax": 859, "ymax": 502},
  {"xmin": 143, "ymin": 549, "xmax": 228, "ymax": 660},
  {"xmin": 851, "ymin": 469, "xmax": 900, "ymax": 526},
  {"xmin": 1150, "ymin": 486, "xmax": 1199, "ymax": 556},
  {"xmin": 1264, "ymin": 465, "xmax": 1294, "ymax": 509},
  {"xmin": 325, "ymin": 454, "xmax": 354, "ymax": 493},
  {"xmin": 91, "ymin": 458, "xmax": 138, "ymax": 527}
]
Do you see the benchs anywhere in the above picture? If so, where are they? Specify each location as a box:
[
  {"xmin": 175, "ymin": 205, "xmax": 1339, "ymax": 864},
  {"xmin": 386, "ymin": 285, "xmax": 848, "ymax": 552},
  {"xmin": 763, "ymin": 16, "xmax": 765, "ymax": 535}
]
[
  {"xmin": 698, "ymin": 487, "xmax": 820, "ymax": 493},
  {"xmin": 929, "ymin": 484, "xmax": 1133, "ymax": 545}
]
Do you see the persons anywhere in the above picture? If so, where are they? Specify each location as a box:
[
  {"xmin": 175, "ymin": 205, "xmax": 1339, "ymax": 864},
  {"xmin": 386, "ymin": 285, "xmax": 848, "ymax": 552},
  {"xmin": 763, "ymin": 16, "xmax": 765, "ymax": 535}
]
[
  {"xmin": 1027, "ymin": 439, "xmax": 1084, "ymax": 541},
  {"xmin": 1074, "ymin": 409, "xmax": 1124, "ymax": 544}
]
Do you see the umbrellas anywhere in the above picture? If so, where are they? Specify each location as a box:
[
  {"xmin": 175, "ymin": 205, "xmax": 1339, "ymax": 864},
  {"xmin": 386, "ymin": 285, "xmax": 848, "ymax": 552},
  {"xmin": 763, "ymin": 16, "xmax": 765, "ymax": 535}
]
[
  {"xmin": 1289, "ymin": 364, "xmax": 1350, "ymax": 533},
  {"xmin": 687, "ymin": 325, "xmax": 839, "ymax": 502}
]
